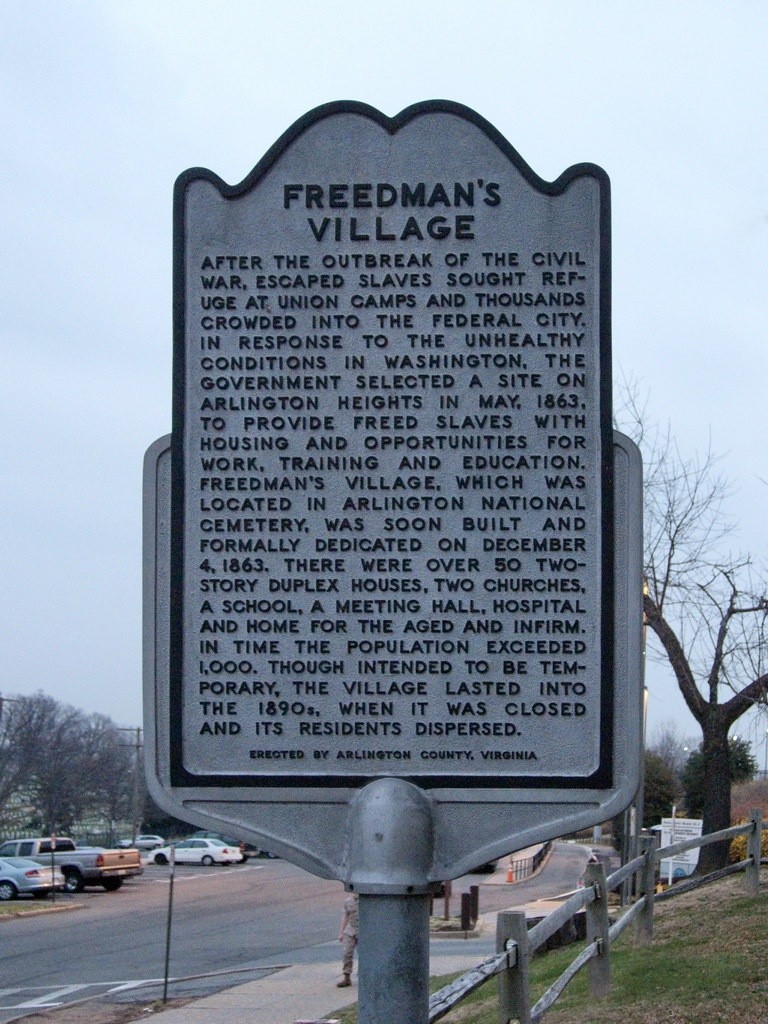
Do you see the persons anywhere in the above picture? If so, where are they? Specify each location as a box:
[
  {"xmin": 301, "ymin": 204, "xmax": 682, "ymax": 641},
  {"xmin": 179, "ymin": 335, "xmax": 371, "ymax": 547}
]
[{"xmin": 337, "ymin": 894, "xmax": 359, "ymax": 987}]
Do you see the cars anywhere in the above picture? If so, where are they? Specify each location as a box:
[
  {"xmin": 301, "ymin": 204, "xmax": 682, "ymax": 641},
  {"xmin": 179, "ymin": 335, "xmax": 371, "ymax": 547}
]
[
  {"xmin": 148, "ymin": 830, "xmax": 259, "ymax": 866},
  {"xmin": 0, "ymin": 857, "xmax": 63, "ymax": 901}
]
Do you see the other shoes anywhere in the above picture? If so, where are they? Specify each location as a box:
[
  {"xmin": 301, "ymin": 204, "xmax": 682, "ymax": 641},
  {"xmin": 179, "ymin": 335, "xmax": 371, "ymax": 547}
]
[{"xmin": 338, "ymin": 979, "xmax": 352, "ymax": 987}]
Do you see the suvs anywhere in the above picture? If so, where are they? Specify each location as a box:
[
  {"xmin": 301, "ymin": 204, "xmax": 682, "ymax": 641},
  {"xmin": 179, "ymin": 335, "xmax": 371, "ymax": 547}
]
[{"xmin": 117, "ymin": 835, "xmax": 163, "ymax": 849}]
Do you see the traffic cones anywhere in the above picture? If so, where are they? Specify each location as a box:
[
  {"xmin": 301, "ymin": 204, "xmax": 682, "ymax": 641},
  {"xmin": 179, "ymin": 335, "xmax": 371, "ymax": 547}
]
[{"xmin": 506, "ymin": 859, "xmax": 515, "ymax": 883}]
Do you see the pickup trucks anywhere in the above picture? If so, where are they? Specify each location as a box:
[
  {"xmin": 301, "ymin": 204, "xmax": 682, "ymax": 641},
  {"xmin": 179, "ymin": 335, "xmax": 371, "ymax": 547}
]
[{"xmin": 0, "ymin": 836, "xmax": 144, "ymax": 894}]
